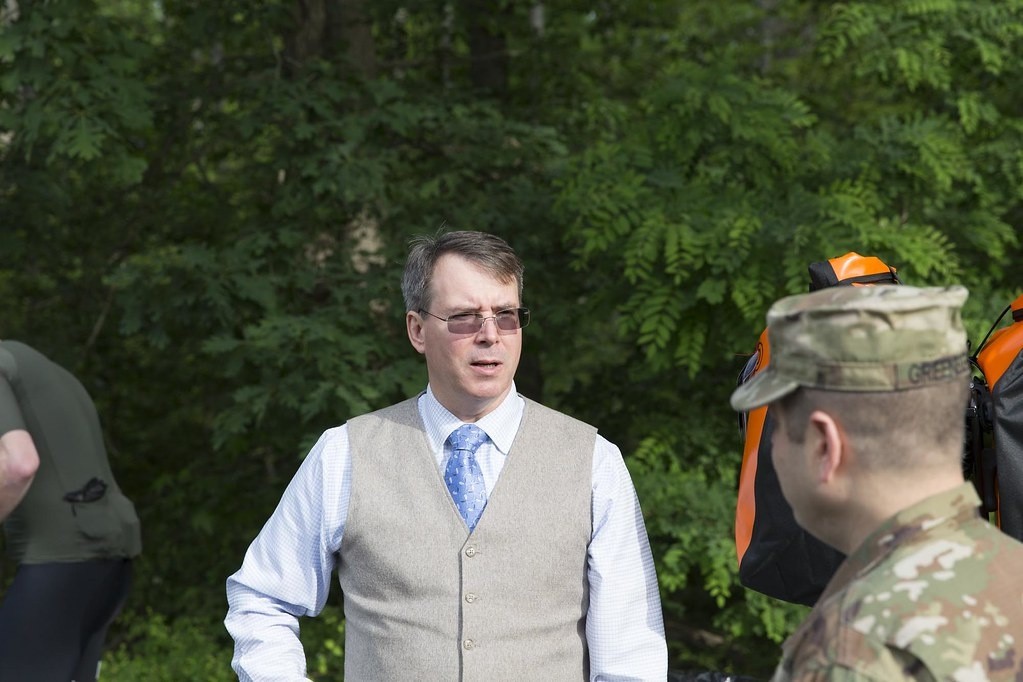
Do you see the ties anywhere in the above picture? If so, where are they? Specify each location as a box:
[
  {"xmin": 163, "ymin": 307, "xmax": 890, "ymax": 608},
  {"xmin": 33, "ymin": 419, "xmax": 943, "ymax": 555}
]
[{"xmin": 444, "ymin": 423, "xmax": 488, "ymax": 533}]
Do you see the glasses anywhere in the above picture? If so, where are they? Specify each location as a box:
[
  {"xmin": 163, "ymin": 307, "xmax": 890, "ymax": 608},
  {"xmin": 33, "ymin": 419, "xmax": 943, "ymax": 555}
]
[{"xmin": 416, "ymin": 307, "xmax": 531, "ymax": 334}]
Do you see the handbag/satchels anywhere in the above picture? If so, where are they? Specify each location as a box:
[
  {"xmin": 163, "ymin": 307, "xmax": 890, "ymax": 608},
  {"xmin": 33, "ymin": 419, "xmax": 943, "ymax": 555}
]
[
  {"xmin": 972, "ymin": 291, "xmax": 1023, "ymax": 543},
  {"xmin": 739, "ymin": 250, "xmax": 994, "ymax": 607}
]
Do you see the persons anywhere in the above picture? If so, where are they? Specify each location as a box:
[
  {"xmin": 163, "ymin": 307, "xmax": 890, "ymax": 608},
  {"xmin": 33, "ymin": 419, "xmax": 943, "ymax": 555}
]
[
  {"xmin": 0, "ymin": 339, "xmax": 143, "ymax": 682},
  {"xmin": 223, "ymin": 231, "xmax": 668, "ymax": 682},
  {"xmin": 728, "ymin": 283, "xmax": 1023, "ymax": 682}
]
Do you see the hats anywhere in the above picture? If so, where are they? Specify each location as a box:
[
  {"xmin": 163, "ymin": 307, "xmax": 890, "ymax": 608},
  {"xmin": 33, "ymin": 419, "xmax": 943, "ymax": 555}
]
[{"xmin": 730, "ymin": 286, "xmax": 970, "ymax": 412}]
